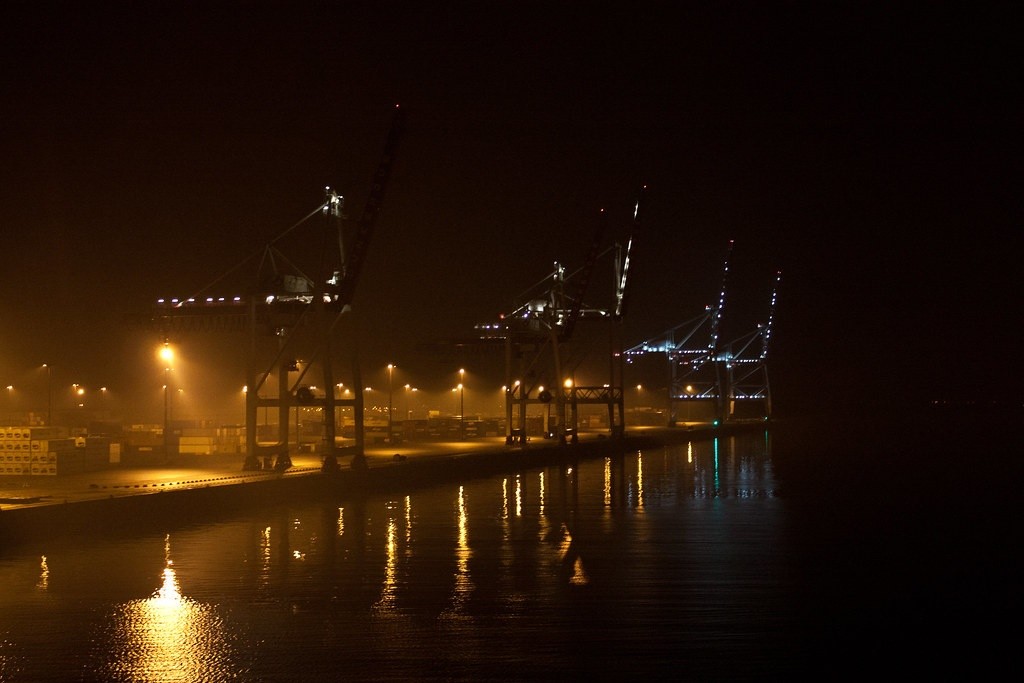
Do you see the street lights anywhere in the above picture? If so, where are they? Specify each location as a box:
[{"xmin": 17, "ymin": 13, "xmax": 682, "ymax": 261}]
[
  {"xmin": 388, "ymin": 364, "xmax": 392, "ymax": 442},
  {"xmin": 686, "ymin": 385, "xmax": 692, "ymax": 417},
  {"xmin": 42, "ymin": 364, "xmax": 51, "ymax": 425},
  {"xmin": 296, "ymin": 386, "xmax": 316, "ymax": 442},
  {"xmin": 338, "ymin": 383, "xmax": 343, "ymax": 425},
  {"xmin": 460, "ymin": 368, "xmax": 464, "ymax": 440},
  {"xmin": 160, "ymin": 336, "xmax": 172, "ymax": 463}
]
